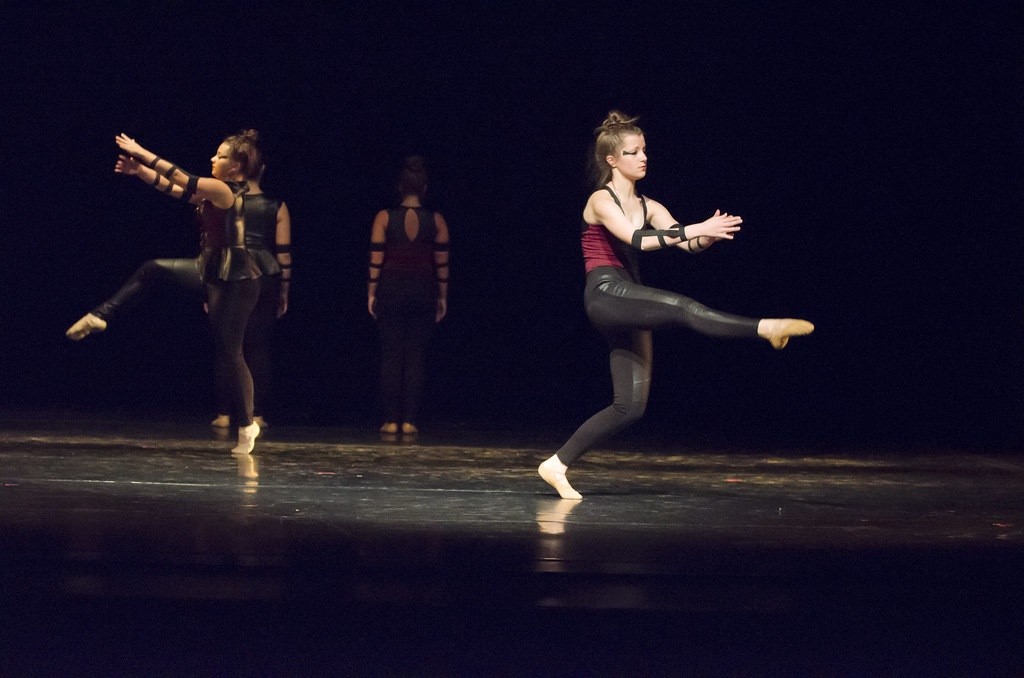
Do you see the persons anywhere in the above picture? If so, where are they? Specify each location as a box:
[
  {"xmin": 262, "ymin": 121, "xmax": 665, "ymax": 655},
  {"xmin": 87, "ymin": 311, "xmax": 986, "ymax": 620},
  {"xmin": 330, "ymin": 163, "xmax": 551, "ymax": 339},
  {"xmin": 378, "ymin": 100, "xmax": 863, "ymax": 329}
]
[
  {"xmin": 66, "ymin": 127, "xmax": 260, "ymax": 455},
  {"xmin": 537, "ymin": 110, "xmax": 815, "ymax": 500},
  {"xmin": 367, "ymin": 156, "xmax": 449, "ymax": 434},
  {"xmin": 208, "ymin": 146, "xmax": 291, "ymax": 428}
]
